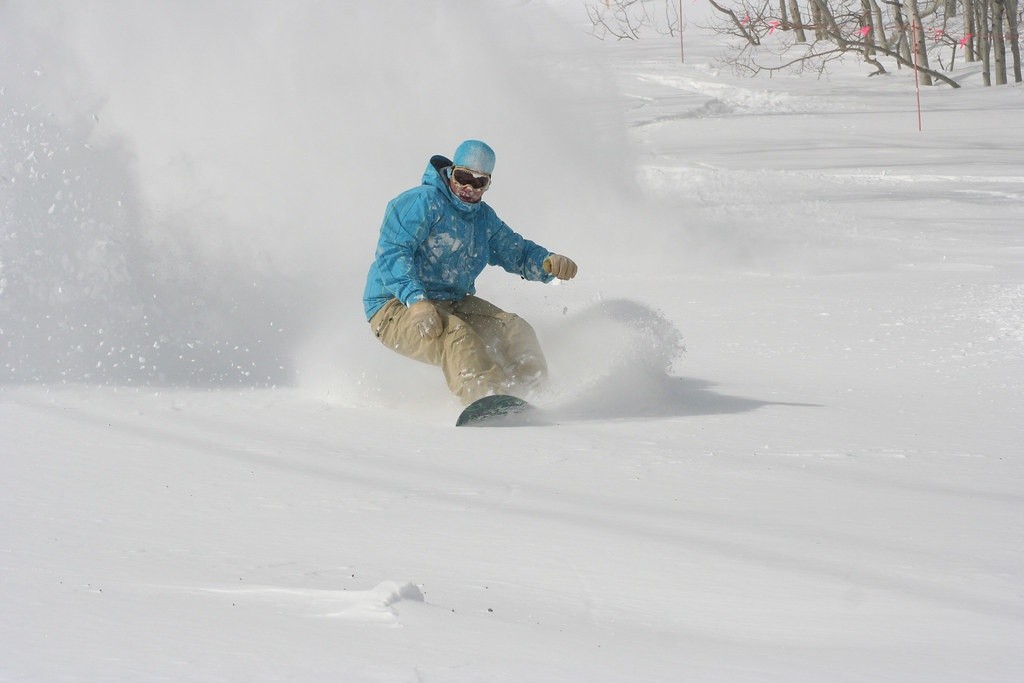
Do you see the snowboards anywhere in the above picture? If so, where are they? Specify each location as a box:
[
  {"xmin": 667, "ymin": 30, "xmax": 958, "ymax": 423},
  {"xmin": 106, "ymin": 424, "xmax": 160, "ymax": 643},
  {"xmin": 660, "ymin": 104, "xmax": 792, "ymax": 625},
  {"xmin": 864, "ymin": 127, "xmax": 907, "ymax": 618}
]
[{"xmin": 455, "ymin": 396, "xmax": 554, "ymax": 426}]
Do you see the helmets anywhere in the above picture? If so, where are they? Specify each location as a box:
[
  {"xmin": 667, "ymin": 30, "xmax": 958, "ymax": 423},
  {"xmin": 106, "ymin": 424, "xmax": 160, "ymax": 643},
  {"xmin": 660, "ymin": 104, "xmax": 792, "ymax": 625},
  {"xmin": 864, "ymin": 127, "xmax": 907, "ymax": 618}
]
[{"xmin": 453, "ymin": 139, "xmax": 496, "ymax": 176}]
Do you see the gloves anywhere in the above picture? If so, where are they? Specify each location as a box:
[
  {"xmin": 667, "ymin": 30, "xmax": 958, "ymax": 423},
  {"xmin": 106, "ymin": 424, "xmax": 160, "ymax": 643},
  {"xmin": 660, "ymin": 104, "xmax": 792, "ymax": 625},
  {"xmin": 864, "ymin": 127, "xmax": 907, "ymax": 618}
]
[
  {"xmin": 409, "ymin": 303, "xmax": 444, "ymax": 340},
  {"xmin": 542, "ymin": 253, "xmax": 577, "ymax": 281}
]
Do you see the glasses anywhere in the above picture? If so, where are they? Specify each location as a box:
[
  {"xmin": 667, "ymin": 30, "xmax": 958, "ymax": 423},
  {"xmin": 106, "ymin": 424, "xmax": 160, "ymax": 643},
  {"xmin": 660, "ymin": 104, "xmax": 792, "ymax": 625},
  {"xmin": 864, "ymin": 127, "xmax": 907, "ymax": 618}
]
[{"xmin": 454, "ymin": 169, "xmax": 489, "ymax": 189}]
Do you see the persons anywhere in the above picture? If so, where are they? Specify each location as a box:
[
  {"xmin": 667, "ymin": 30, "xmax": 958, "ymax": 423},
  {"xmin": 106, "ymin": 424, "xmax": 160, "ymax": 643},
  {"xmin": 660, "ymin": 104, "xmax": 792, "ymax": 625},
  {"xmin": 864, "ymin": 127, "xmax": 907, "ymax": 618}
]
[{"xmin": 363, "ymin": 140, "xmax": 578, "ymax": 409}]
